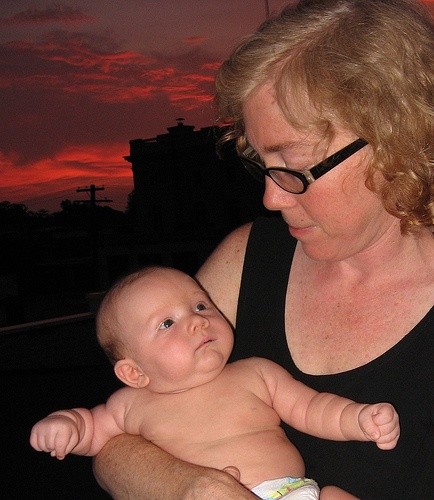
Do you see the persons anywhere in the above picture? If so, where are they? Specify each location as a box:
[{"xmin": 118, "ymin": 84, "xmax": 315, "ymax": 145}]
[
  {"xmin": 94, "ymin": 0, "xmax": 434, "ymax": 500},
  {"xmin": 31, "ymin": 267, "xmax": 401, "ymax": 500}
]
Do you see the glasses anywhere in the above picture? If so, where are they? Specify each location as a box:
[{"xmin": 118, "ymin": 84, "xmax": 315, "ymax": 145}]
[{"xmin": 240, "ymin": 138, "xmax": 369, "ymax": 195}]
